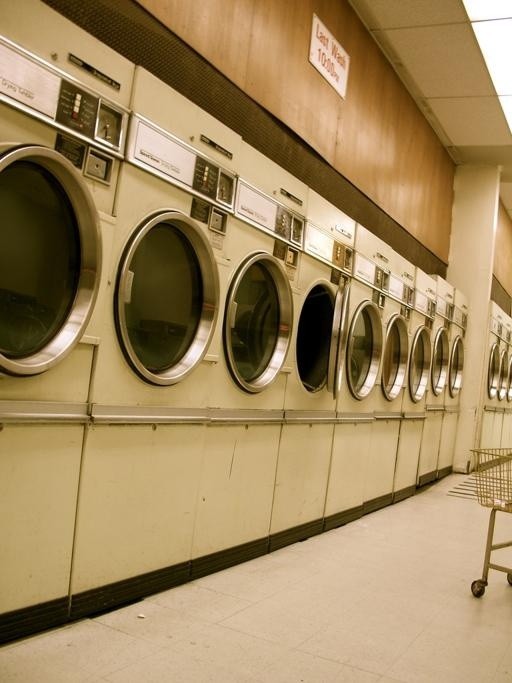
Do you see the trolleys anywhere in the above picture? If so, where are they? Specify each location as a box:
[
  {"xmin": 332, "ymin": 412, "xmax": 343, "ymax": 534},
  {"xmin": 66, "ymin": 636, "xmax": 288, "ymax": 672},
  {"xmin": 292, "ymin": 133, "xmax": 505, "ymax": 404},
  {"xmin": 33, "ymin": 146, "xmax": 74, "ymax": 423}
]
[{"xmin": 469, "ymin": 444, "xmax": 512, "ymax": 598}]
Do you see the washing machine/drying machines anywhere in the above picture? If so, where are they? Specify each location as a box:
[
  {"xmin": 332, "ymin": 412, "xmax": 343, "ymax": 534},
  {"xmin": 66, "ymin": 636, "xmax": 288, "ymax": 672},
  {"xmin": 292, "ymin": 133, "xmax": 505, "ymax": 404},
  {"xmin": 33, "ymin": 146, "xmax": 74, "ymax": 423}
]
[
  {"xmin": 476, "ymin": 301, "xmax": 512, "ymax": 468},
  {"xmin": 1, "ymin": 0, "xmax": 468, "ymax": 646}
]
